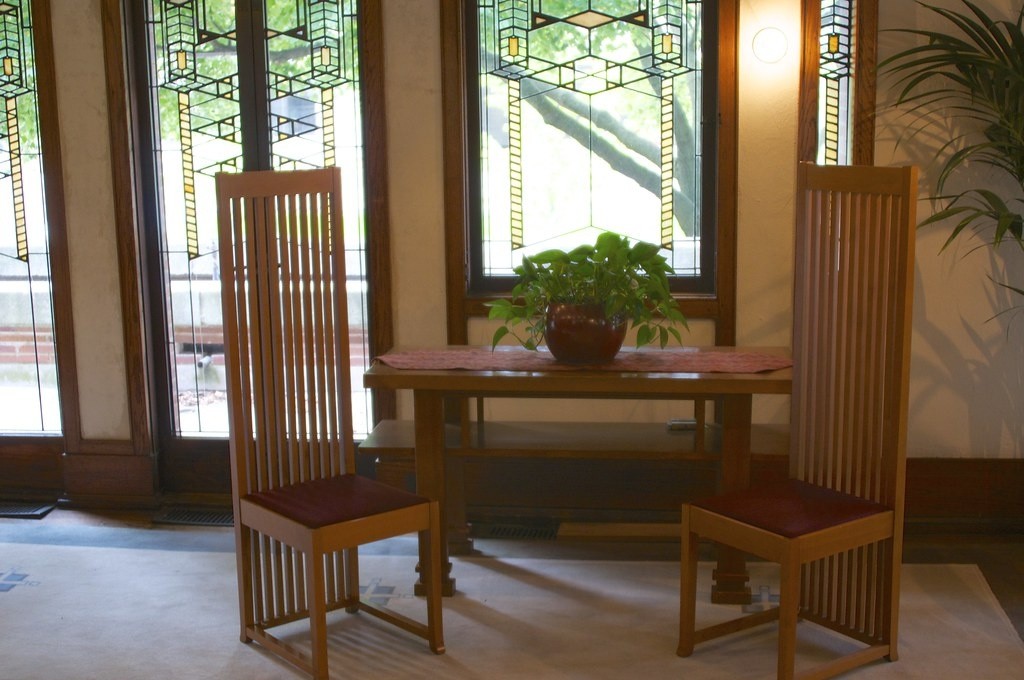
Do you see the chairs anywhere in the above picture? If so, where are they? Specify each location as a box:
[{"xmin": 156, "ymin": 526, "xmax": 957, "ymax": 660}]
[
  {"xmin": 677, "ymin": 160, "xmax": 919, "ymax": 680},
  {"xmin": 216, "ymin": 166, "xmax": 446, "ymax": 679}
]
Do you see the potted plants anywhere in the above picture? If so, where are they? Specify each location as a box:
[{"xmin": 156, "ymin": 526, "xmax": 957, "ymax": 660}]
[{"xmin": 483, "ymin": 231, "xmax": 690, "ymax": 362}]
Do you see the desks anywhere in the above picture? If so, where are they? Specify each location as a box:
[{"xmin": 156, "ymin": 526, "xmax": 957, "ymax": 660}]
[{"xmin": 362, "ymin": 344, "xmax": 793, "ymax": 604}]
